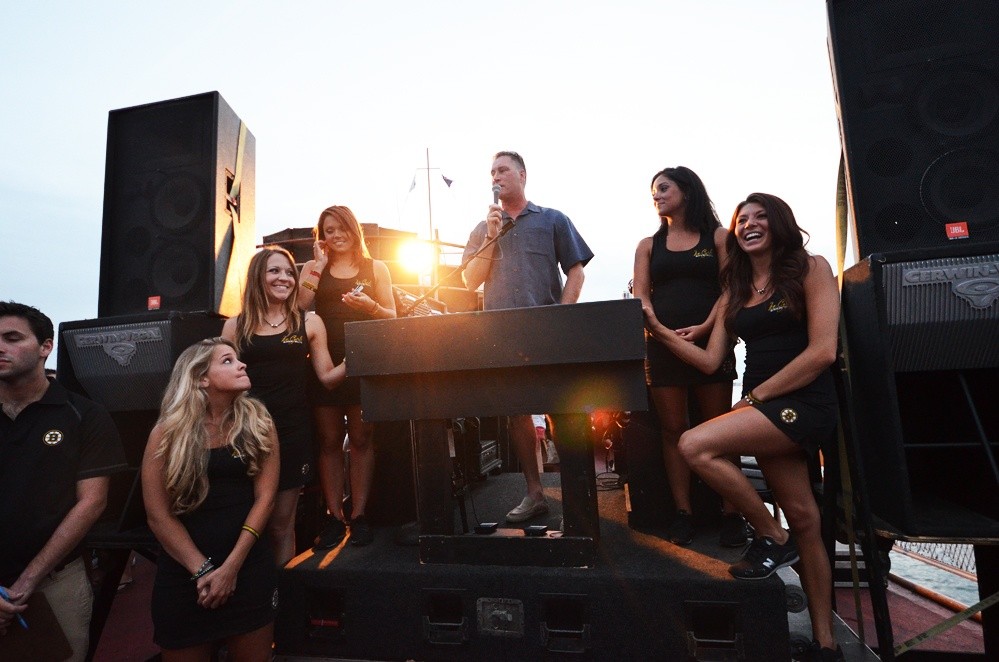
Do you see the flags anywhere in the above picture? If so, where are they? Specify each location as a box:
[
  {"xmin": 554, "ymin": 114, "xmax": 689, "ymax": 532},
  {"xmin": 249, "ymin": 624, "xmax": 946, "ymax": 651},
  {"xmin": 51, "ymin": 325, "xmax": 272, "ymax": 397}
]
[
  {"xmin": 442, "ymin": 174, "xmax": 452, "ymax": 187},
  {"xmin": 408, "ymin": 175, "xmax": 416, "ymax": 192}
]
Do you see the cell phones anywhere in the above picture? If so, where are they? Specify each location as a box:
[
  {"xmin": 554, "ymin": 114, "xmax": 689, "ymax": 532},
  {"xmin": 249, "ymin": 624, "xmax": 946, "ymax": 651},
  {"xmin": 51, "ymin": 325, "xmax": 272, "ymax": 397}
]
[{"xmin": 342, "ymin": 285, "xmax": 364, "ymax": 303}]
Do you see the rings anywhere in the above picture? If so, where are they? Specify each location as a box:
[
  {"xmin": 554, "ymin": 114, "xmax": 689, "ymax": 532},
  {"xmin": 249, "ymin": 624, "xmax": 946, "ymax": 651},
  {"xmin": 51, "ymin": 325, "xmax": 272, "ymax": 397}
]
[{"xmin": 312, "ymin": 246, "xmax": 315, "ymax": 247}]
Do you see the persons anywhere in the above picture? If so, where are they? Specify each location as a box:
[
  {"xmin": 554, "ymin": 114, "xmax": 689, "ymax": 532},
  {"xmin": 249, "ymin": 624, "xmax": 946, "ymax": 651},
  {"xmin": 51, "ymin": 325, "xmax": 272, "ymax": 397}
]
[
  {"xmin": 0, "ymin": 301, "xmax": 129, "ymax": 662},
  {"xmin": 300, "ymin": 205, "xmax": 397, "ymax": 548},
  {"xmin": 141, "ymin": 337, "xmax": 281, "ymax": 662},
  {"xmin": 219, "ymin": 245, "xmax": 347, "ymax": 570},
  {"xmin": 678, "ymin": 192, "xmax": 842, "ymax": 662},
  {"xmin": 462, "ymin": 151, "xmax": 595, "ymax": 532},
  {"xmin": 632, "ymin": 166, "xmax": 749, "ymax": 549}
]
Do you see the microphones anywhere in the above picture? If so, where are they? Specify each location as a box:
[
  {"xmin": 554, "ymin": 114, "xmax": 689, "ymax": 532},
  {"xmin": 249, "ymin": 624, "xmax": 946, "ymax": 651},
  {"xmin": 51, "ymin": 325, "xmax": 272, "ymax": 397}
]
[{"xmin": 492, "ymin": 184, "xmax": 503, "ymax": 225}]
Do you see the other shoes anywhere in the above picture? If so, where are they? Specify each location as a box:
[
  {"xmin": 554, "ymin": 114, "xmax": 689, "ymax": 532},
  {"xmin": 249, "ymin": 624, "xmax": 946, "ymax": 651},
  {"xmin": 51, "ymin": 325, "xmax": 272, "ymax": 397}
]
[{"xmin": 505, "ymin": 497, "xmax": 549, "ymax": 522}]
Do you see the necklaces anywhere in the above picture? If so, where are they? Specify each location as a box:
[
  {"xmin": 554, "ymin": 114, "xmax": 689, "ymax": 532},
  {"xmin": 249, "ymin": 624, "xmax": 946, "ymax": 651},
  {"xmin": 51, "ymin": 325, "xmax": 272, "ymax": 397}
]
[
  {"xmin": 750, "ymin": 274, "xmax": 773, "ymax": 293},
  {"xmin": 263, "ymin": 316, "xmax": 286, "ymax": 328}
]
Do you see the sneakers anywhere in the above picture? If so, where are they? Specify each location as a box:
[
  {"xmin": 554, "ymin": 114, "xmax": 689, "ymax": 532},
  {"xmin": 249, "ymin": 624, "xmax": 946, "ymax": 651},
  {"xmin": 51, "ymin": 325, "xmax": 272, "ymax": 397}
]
[
  {"xmin": 348, "ymin": 516, "xmax": 372, "ymax": 547},
  {"xmin": 728, "ymin": 530, "xmax": 799, "ymax": 580},
  {"xmin": 670, "ymin": 510, "xmax": 694, "ymax": 544},
  {"xmin": 718, "ymin": 513, "xmax": 748, "ymax": 547},
  {"xmin": 792, "ymin": 639, "xmax": 844, "ymax": 662},
  {"xmin": 318, "ymin": 516, "xmax": 345, "ymax": 547}
]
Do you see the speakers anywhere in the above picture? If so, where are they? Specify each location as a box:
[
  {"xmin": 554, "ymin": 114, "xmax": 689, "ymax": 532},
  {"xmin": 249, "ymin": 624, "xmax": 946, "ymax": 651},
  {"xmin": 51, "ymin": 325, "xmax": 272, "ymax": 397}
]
[
  {"xmin": 826, "ymin": 0, "xmax": 999, "ymax": 260},
  {"xmin": 833, "ymin": 242, "xmax": 999, "ymax": 538},
  {"xmin": 55, "ymin": 312, "xmax": 226, "ymax": 465},
  {"xmin": 97, "ymin": 90, "xmax": 255, "ymax": 317}
]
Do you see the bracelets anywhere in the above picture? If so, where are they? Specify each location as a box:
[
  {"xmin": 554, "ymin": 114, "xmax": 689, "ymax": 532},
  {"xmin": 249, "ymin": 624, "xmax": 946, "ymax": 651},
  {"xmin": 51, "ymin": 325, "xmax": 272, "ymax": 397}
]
[
  {"xmin": 368, "ymin": 302, "xmax": 380, "ymax": 316},
  {"xmin": 743, "ymin": 391, "xmax": 764, "ymax": 405},
  {"xmin": 190, "ymin": 556, "xmax": 215, "ymax": 582},
  {"xmin": 241, "ymin": 524, "xmax": 260, "ymax": 541},
  {"xmin": 343, "ymin": 357, "xmax": 347, "ymax": 362},
  {"xmin": 309, "ymin": 270, "xmax": 322, "ymax": 279},
  {"xmin": 302, "ymin": 280, "xmax": 318, "ymax": 294},
  {"xmin": 484, "ymin": 232, "xmax": 497, "ymax": 242}
]
